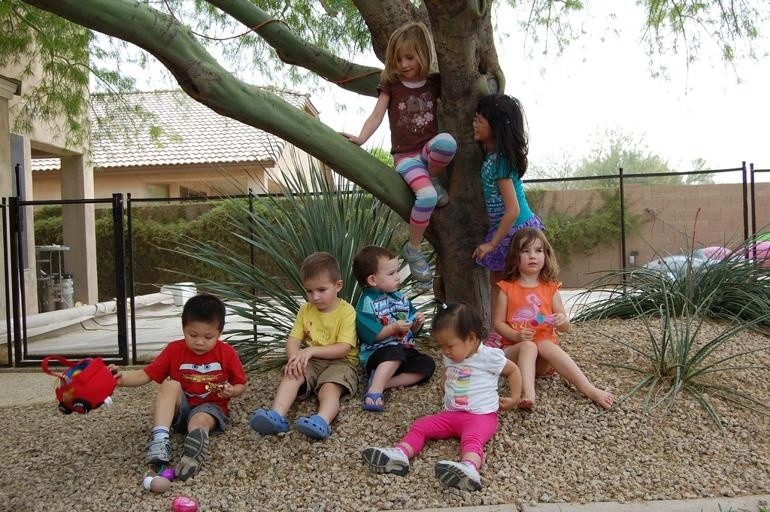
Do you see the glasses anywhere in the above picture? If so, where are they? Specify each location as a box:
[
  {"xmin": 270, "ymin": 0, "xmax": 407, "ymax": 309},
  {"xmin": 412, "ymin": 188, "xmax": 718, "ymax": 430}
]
[{"xmin": 471, "ymin": 114, "xmax": 487, "ymax": 128}]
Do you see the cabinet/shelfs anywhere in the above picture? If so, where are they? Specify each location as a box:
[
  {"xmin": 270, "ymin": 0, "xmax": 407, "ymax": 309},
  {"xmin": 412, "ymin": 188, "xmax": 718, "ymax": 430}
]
[{"xmin": 36, "ymin": 246, "xmax": 70, "ymax": 308}]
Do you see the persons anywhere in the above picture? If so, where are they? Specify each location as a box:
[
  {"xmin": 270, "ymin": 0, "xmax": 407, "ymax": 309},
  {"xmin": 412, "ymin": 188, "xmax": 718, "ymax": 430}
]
[
  {"xmin": 362, "ymin": 298, "xmax": 521, "ymax": 492},
  {"xmin": 249, "ymin": 252, "xmax": 356, "ymax": 439},
  {"xmin": 492, "ymin": 227, "xmax": 614, "ymax": 411},
  {"xmin": 471, "ymin": 94, "xmax": 545, "ymax": 309},
  {"xmin": 353, "ymin": 246, "xmax": 436, "ymax": 411},
  {"xmin": 106, "ymin": 293, "xmax": 247, "ymax": 481},
  {"xmin": 337, "ymin": 21, "xmax": 458, "ymax": 283}
]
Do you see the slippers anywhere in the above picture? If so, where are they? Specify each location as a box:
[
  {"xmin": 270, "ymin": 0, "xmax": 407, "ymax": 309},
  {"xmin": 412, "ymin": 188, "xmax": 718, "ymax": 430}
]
[
  {"xmin": 250, "ymin": 409, "xmax": 290, "ymax": 434},
  {"xmin": 362, "ymin": 393, "xmax": 385, "ymax": 412},
  {"xmin": 296, "ymin": 414, "xmax": 331, "ymax": 440}
]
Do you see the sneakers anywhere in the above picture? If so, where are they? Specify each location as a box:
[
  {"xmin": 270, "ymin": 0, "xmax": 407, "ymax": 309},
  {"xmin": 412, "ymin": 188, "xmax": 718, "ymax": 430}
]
[
  {"xmin": 435, "ymin": 460, "xmax": 482, "ymax": 492},
  {"xmin": 362, "ymin": 447, "xmax": 410, "ymax": 477},
  {"xmin": 431, "ymin": 177, "xmax": 450, "ymax": 208},
  {"xmin": 174, "ymin": 428, "xmax": 210, "ymax": 481},
  {"xmin": 144, "ymin": 438, "xmax": 172, "ymax": 466},
  {"xmin": 402, "ymin": 243, "xmax": 432, "ymax": 283}
]
